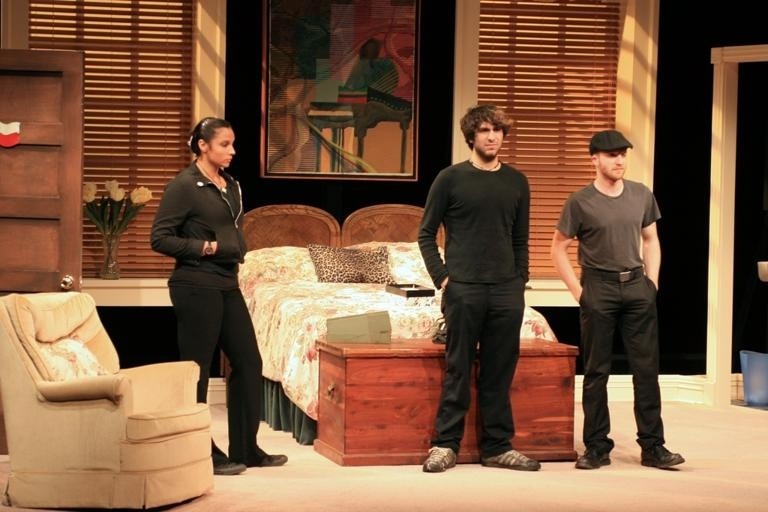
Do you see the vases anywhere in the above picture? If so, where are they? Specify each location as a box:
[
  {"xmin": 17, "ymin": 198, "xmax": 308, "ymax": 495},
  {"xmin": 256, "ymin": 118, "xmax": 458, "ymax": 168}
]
[{"xmin": 98, "ymin": 230, "xmax": 121, "ymax": 278}]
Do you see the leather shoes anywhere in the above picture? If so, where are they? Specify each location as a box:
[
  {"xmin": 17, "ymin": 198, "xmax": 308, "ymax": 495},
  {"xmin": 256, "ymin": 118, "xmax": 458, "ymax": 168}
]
[
  {"xmin": 641, "ymin": 446, "xmax": 685, "ymax": 468},
  {"xmin": 575, "ymin": 449, "xmax": 610, "ymax": 469}
]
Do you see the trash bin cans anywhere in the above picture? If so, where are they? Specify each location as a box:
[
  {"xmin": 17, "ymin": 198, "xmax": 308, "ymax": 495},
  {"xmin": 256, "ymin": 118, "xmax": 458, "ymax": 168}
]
[{"xmin": 741, "ymin": 350, "xmax": 768, "ymax": 405}]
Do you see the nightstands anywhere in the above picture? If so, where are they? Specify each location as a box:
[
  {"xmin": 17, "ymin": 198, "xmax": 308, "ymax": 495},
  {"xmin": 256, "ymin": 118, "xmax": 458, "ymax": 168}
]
[{"xmin": 83, "ymin": 276, "xmax": 173, "ymax": 310}]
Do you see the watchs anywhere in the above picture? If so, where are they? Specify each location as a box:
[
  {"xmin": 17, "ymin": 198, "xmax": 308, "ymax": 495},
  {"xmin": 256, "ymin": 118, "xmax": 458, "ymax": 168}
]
[{"xmin": 205, "ymin": 240, "xmax": 212, "ymax": 256}]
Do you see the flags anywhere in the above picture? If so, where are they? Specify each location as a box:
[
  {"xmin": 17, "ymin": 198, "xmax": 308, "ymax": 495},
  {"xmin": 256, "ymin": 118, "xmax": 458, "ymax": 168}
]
[{"xmin": 0, "ymin": 121, "xmax": 20, "ymax": 147}]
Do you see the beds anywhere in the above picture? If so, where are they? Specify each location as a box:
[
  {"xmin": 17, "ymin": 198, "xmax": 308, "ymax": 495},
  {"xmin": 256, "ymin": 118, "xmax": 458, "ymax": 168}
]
[{"xmin": 219, "ymin": 203, "xmax": 559, "ymax": 446}]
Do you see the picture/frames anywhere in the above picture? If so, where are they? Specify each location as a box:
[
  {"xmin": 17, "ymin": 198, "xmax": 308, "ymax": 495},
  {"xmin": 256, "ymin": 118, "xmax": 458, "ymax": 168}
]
[{"xmin": 260, "ymin": 0, "xmax": 421, "ymax": 183}]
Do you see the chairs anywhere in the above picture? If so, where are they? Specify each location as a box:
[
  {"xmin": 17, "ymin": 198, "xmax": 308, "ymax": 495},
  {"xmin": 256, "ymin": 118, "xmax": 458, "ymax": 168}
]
[{"xmin": 0, "ymin": 291, "xmax": 214, "ymax": 512}]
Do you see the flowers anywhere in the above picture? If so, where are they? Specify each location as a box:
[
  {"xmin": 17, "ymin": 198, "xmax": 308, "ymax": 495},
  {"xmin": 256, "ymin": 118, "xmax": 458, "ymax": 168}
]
[{"xmin": 82, "ymin": 180, "xmax": 153, "ymax": 205}]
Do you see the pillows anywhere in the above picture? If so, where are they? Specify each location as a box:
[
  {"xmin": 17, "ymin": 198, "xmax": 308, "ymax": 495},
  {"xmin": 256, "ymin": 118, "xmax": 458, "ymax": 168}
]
[
  {"xmin": 308, "ymin": 243, "xmax": 396, "ymax": 285},
  {"xmin": 40, "ymin": 339, "xmax": 108, "ymax": 384}
]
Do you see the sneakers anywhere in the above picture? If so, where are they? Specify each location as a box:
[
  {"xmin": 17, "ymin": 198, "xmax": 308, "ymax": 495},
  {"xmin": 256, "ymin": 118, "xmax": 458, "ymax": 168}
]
[
  {"xmin": 482, "ymin": 450, "xmax": 540, "ymax": 470},
  {"xmin": 259, "ymin": 455, "xmax": 288, "ymax": 466},
  {"xmin": 424, "ymin": 446, "xmax": 457, "ymax": 472},
  {"xmin": 214, "ymin": 463, "xmax": 246, "ymax": 475}
]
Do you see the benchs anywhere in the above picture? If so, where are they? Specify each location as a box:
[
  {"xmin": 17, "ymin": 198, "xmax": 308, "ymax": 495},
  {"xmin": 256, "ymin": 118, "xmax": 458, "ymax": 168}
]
[{"xmin": 315, "ymin": 337, "xmax": 580, "ymax": 467}]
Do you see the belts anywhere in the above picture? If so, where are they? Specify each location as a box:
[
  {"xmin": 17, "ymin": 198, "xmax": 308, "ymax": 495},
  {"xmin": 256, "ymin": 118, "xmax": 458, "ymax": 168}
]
[{"xmin": 583, "ymin": 268, "xmax": 643, "ymax": 282}]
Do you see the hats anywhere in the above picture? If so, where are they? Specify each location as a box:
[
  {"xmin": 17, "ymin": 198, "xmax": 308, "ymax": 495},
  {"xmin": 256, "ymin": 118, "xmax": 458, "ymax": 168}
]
[{"xmin": 590, "ymin": 131, "xmax": 632, "ymax": 154}]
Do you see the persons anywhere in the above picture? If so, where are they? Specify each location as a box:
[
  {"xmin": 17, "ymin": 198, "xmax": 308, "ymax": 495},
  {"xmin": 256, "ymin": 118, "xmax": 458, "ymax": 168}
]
[
  {"xmin": 551, "ymin": 130, "xmax": 686, "ymax": 471},
  {"xmin": 151, "ymin": 118, "xmax": 288, "ymax": 474},
  {"xmin": 418, "ymin": 106, "xmax": 540, "ymax": 471}
]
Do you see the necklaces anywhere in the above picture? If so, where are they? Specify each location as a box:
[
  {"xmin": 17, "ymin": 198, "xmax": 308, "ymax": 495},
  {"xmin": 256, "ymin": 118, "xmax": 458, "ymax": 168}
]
[
  {"xmin": 195, "ymin": 159, "xmax": 230, "ymax": 194},
  {"xmin": 468, "ymin": 158, "xmax": 499, "ymax": 173}
]
